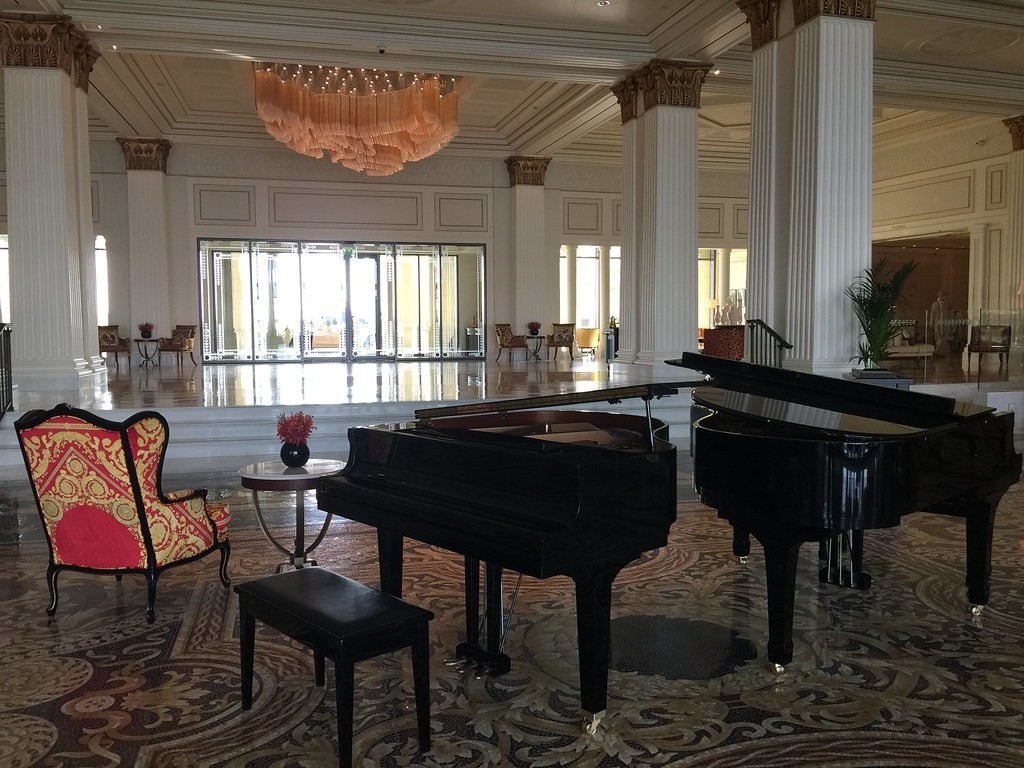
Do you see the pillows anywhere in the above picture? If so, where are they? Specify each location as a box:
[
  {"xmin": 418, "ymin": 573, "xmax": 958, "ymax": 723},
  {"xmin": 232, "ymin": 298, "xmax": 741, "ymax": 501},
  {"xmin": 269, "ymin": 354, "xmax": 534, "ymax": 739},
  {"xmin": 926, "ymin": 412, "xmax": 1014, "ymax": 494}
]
[
  {"xmin": 171, "ymin": 329, "xmax": 191, "ymax": 345},
  {"xmin": 498, "ymin": 328, "xmax": 512, "ymax": 341},
  {"xmin": 555, "ymin": 327, "xmax": 572, "ymax": 342},
  {"xmin": 99, "ymin": 330, "xmax": 121, "ymax": 346}
]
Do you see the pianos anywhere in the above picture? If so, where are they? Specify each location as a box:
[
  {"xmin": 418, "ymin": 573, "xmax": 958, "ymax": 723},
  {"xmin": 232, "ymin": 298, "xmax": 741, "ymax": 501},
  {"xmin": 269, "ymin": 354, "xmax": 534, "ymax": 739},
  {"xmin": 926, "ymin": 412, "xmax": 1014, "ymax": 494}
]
[
  {"xmin": 663, "ymin": 352, "xmax": 1024, "ymax": 685},
  {"xmin": 315, "ymin": 379, "xmax": 712, "ymax": 737}
]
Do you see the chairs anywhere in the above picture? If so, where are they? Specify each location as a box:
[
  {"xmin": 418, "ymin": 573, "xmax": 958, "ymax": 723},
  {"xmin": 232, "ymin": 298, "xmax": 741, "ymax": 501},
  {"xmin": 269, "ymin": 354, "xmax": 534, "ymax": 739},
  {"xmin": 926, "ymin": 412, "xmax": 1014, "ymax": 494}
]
[
  {"xmin": 159, "ymin": 324, "xmax": 198, "ymax": 368},
  {"xmin": 546, "ymin": 323, "xmax": 577, "ymax": 361},
  {"xmin": 493, "ymin": 323, "xmax": 529, "ymax": 360},
  {"xmin": 703, "ymin": 328, "xmax": 746, "ymax": 361},
  {"xmin": 99, "ymin": 324, "xmax": 130, "ymax": 367},
  {"xmin": 14, "ymin": 404, "xmax": 231, "ymax": 623}
]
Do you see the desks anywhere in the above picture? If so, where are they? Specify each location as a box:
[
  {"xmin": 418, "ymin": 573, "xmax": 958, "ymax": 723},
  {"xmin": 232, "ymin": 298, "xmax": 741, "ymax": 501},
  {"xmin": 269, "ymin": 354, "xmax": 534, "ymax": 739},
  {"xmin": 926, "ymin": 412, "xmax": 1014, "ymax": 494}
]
[
  {"xmin": 237, "ymin": 456, "xmax": 351, "ymax": 573},
  {"xmin": 134, "ymin": 338, "xmax": 159, "ymax": 367},
  {"xmin": 523, "ymin": 335, "xmax": 546, "ymax": 361}
]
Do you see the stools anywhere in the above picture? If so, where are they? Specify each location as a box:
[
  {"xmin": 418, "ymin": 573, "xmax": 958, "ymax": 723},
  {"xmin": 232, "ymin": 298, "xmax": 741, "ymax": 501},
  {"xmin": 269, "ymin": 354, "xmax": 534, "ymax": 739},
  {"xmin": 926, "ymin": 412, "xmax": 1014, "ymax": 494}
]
[{"xmin": 233, "ymin": 565, "xmax": 434, "ymax": 767}]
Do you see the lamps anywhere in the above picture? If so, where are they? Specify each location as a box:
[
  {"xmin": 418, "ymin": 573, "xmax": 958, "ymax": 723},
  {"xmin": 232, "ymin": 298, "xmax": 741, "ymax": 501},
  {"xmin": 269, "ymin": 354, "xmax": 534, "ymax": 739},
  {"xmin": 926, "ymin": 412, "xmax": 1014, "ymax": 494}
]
[{"xmin": 258, "ymin": 68, "xmax": 461, "ymax": 178}]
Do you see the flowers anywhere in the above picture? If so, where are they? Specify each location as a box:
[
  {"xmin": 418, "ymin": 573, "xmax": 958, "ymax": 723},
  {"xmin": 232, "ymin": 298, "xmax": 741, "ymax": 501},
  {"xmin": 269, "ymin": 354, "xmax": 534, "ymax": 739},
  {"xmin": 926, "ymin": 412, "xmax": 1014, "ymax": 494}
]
[
  {"xmin": 278, "ymin": 410, "xmax": 313, "ymax": 444},
  {"xmin": 138, "ymin": 321, "xmax": 154, "ymax": 331},
  {"xmin": 528, "ymin": 321, "xmax": 542, "ymax": 329}
]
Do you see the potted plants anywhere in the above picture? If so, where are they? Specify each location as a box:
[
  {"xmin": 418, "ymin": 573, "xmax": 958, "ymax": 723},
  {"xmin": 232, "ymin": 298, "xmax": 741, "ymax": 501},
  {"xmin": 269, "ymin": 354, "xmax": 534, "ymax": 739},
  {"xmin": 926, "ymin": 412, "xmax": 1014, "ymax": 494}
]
[{"xmin": 843, "ymin": 252, "xmax": 918, "ymax": 390}]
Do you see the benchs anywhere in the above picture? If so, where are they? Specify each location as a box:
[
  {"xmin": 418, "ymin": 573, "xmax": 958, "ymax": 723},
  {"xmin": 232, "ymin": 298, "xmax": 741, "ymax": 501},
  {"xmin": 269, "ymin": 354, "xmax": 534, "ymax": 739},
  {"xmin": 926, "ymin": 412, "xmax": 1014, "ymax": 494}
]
[{"xmin": 968, "ymin": 325, "xmax": 1012, "ymax": 364}]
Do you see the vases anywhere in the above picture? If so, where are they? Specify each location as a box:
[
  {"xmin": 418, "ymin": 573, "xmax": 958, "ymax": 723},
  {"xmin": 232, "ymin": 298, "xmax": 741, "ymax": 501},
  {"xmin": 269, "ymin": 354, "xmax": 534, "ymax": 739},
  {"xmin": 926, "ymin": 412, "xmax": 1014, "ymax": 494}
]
[
  {"xmin": 530, "ymin": 328, "xmax": 539, "ymax": 335},
  {"xmin": 141, "ymin": 330, "xmax": 152, "ymax": 338},
  {"xmin": 278, "ymin": 440, "xmax": 311, "ymax": 469}
]
